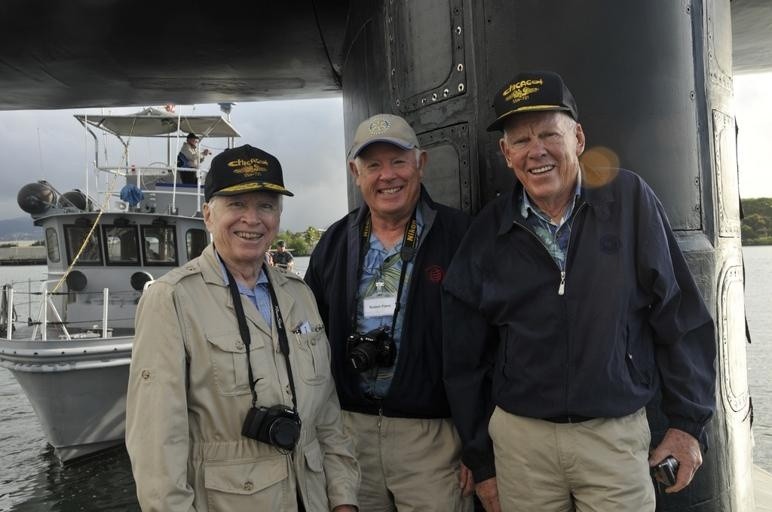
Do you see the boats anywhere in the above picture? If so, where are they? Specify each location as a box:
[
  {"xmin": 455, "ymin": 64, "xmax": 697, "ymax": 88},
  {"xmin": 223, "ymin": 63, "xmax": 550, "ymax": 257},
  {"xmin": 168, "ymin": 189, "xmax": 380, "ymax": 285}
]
[{"xmin": 0, "ymin": 102, "xmax": 298, "ymax": 467}]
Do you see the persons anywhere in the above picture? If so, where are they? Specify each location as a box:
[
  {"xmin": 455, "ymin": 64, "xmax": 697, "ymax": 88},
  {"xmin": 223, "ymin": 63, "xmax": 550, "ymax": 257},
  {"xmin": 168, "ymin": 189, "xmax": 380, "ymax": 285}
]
[
  {"xmin": 126, "ymin": 143, "xmax": 361, "ymax": 512},
  {"xmin": 303, "ymin": 115, "xmax": 477, "ymax": 511},
  {"xmin": 440, "ymin": 67, "xmax": 717, "ymax": 511},
  {"xmin": 271, "ymin": 240, "xmax": 295, "ymax": 271},
  {"xmin": 177, "ymin": 132, "xmax": 212, "ymax": 187}
]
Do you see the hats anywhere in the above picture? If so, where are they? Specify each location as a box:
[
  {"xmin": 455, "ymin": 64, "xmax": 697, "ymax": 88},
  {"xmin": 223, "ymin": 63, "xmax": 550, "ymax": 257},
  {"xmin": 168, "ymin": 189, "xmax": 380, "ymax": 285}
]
[
  {"xmin": 205, "ymin": 142, "xmax": 295, "ymax": 205},
  {"xmin": 276, "ymin": 240, "xmax": 285, "ymax": 247},
  {"xmin": 485, "ymin": 69, "xmax": 581, "ymax": 131},
  {"xmin": 348, "ymin": 113, "xmax": 423, "ymax": 161}
]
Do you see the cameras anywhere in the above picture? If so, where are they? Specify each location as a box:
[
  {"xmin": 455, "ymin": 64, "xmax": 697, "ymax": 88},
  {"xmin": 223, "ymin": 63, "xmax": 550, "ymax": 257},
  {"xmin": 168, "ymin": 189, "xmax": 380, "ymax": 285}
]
[
  {"xmin": 655, "ymin": 457, "xmax": 679, "ymax": 487},
  {"xmin": 241, "ymin": 404, "xmax": 302, "ymax": 452},
  {"xmin": 346, "ymin": 329, "xmax": 397, "ymax": 369}
]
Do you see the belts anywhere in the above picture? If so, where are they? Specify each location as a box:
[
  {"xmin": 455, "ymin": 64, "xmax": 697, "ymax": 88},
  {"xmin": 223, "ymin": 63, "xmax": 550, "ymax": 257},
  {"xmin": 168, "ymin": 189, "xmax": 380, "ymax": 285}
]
[{"xmin": 338, "ymin": 398, "xmax": 453, "ymax": 422}]
